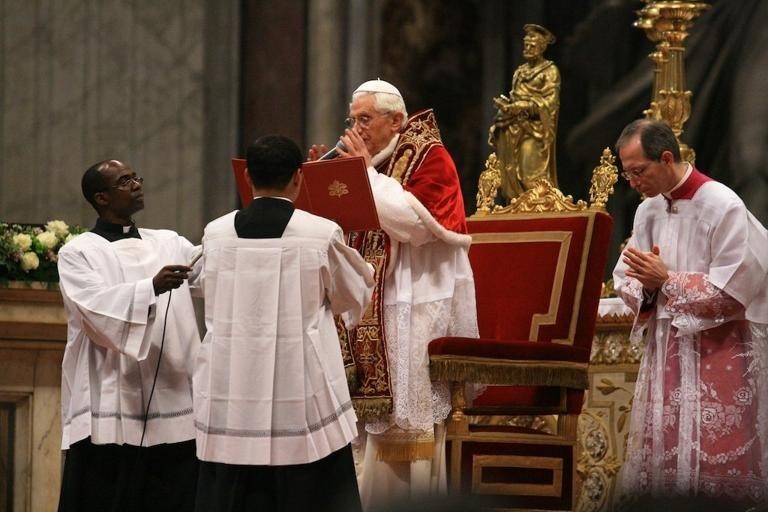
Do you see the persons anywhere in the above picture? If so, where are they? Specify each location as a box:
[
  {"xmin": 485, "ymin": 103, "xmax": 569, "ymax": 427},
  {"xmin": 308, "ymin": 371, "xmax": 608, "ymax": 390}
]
[
  {"xmin": 55, "ymin": 156, "xmax": 205, "ymax": 512},
  {"xmin": 306, "ymin": 77, "xmax": 487, "ymax": 512},
  {"xmin": 611, "ymin": 117, "xmax": 768, "ymax": 511},
  {"xmin": 487, "ymin": 21, "xmax": 559, "ymax": 201},
  {"xmin": 180, "ymin": 133, "xmax": 380, "ymax": 512}
]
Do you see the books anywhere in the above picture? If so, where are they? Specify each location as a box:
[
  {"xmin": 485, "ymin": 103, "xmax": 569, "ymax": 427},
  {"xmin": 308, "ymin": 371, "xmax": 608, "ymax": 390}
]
[{"xmin": 231, "ymin": 156, "xmax": 380, "ymax": 234}]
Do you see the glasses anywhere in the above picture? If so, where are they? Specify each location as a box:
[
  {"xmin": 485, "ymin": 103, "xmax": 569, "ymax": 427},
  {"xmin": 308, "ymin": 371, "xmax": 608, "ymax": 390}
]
[
  {"xmin": 621, "ymin": 159, "xmax": 654, "ymax": 181},
  {"xmin": 101, "ymin": 176, "xmax": 143, "ymax": 194},
  {"xmin": 343, "ymin": 111, "xmax": 390, "ymax": 129}
]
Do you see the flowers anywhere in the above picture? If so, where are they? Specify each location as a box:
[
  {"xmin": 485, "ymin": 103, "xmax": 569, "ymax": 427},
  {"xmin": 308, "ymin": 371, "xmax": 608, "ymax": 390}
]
[{"xmin": 1, "ymin": 216, "xmax": 87, "ymax": 289}]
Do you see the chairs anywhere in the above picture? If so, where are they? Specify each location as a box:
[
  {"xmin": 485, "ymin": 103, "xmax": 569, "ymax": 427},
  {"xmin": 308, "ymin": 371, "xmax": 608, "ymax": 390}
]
[{"xmin": 378, "ymin": 143, "xmax": 620, "ymax": 509}]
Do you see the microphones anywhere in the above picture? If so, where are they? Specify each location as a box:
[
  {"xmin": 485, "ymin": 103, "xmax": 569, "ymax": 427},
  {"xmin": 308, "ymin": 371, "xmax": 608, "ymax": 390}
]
[{"xmin": 317, "ymin": 140, "xmax": 346, "ymax": 161}]
